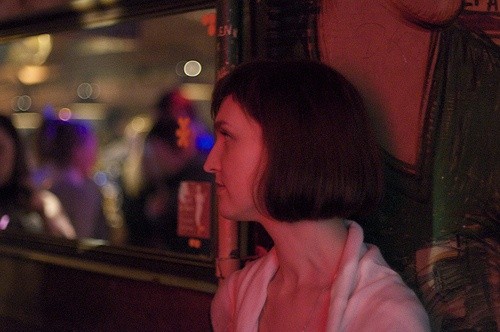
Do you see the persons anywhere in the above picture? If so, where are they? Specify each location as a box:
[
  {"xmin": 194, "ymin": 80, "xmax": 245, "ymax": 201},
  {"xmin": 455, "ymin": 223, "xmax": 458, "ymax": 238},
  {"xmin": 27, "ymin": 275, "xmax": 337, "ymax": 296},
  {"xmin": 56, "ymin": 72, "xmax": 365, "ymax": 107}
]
[
  {"xmin": 36, "ymin": 118, "xmax": 113, "ymax": 244},
  {"xmin": 0, "ymin": 112, "xmax": 77, "ymax": 239},
  {"xmin": 138, "ymin": 90, "xmax": 212, "ymax": 250},
  {"xmin": 203, "ymin": 61, "xmax": 432, "ymax": 332}
]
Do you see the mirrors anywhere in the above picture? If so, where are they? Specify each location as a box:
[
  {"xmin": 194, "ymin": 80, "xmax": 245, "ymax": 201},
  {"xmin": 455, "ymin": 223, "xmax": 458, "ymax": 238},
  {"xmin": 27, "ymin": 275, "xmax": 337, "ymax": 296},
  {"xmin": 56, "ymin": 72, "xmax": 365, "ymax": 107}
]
[{"xmin": 1, "ymin": 0, "xmax": 254, "ymax": 294}]
